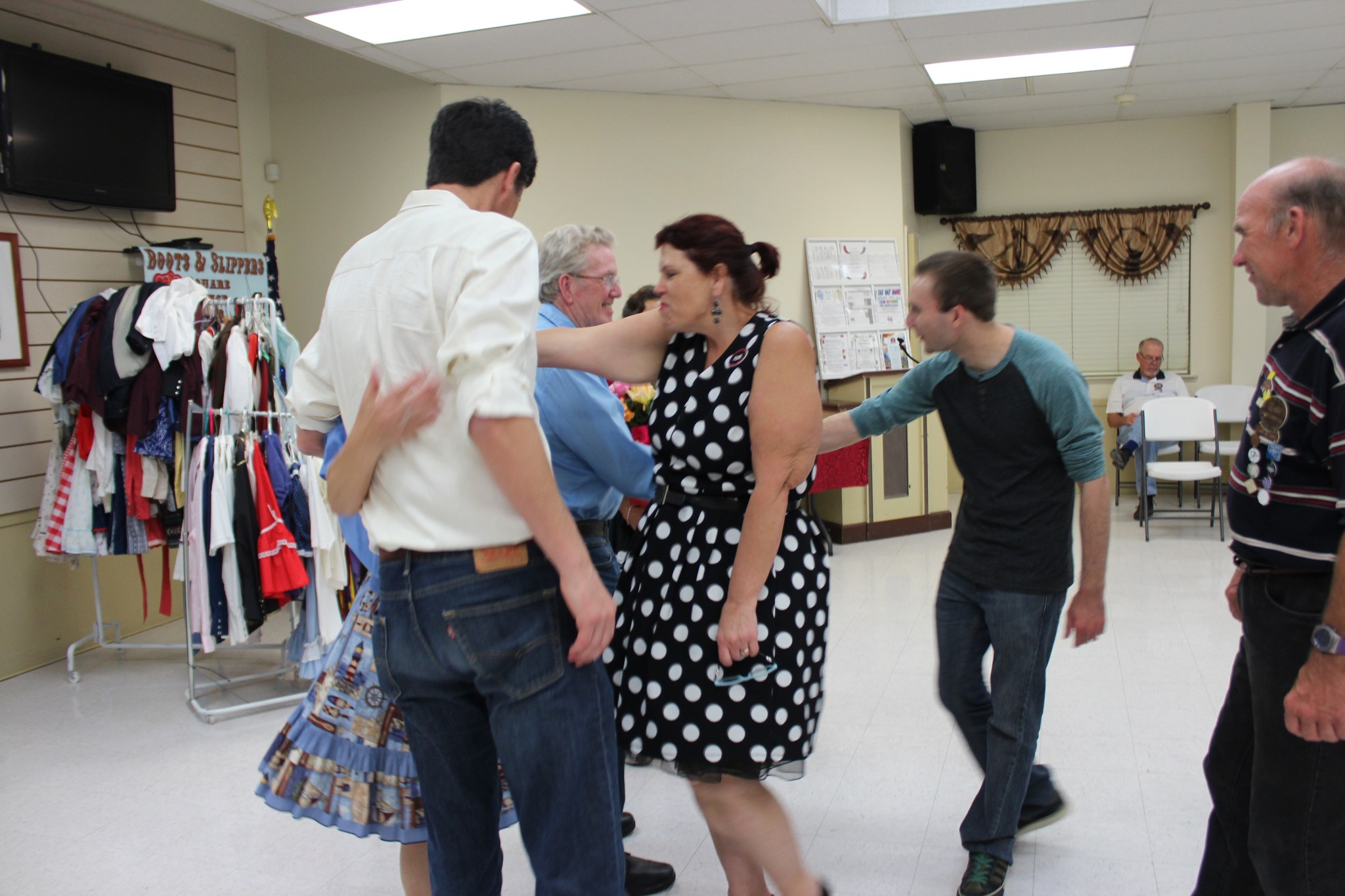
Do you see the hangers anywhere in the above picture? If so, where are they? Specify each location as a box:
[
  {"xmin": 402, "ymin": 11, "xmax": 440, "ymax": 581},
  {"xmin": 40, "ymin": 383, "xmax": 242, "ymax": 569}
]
[
  {"xmin": 202, "ymin": 296, "xmax": 282, "ymax": 335},
  {"xmin": 201, "ymin": 402, "xmax": 307, "ymax": 468}
]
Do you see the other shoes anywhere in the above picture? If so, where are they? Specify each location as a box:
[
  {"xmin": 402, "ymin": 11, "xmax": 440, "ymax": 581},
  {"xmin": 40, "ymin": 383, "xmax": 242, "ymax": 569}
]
[
  {"xmin": 1133, "ymin": 498, "xmax": 1153, "ymax": 519},
  {"xmin": 1110, "ymin": 448, "xmax": 1131, "ymax": 470}
]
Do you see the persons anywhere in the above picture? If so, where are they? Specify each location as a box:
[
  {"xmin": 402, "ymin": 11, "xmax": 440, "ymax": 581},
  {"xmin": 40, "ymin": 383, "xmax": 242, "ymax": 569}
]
[
  {"xmin": 528, "ymin": 211, "xmax": 841, "ymax": 896},
  {"xmin": 511, "ymin": 220, "xmax": 681, "ymax": 891},
  {"xmin": 820, "ymin": 245, "xmax": 1121, "ymax": 896},
  {"xmin": 1106, "ymin": 337, "xmax": 1190, "ymax": 523},
  {"xmin": 317, "ymin": 359, "xmax": 518, "ymax": 896},
  {"xmin": 606, "ymin": 280, "xmax": 680, "ymax": 526},
  {"xmin": 292, "ymin": 91, "xmax": 647, "ymax": 895},
  {"xmin": 1169, "ymin": 151, "xmax": 1344, "ymax": 896}
]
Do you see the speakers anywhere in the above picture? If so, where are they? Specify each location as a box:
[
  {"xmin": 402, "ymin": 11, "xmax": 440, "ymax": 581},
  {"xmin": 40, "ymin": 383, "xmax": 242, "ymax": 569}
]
[{"xmin": 913, "ymin": 117, "xmax": 976, "ymax": 215}]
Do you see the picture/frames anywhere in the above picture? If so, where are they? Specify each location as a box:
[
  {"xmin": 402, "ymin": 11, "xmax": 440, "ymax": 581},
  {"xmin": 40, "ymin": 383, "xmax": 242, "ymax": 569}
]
[{"xmin": 0, "ymin": 224, "xmax": 31, "ymax": 371}]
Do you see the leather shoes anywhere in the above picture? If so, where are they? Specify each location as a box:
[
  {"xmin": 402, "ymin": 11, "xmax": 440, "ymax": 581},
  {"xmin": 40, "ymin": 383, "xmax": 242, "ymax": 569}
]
[
  {"xmin": 623, "ymin": 851, "xmax": 675, "ymax": 896},
  {"xmin": 620, "ymin": 811, "xmax": 635, "ymax": 836}
]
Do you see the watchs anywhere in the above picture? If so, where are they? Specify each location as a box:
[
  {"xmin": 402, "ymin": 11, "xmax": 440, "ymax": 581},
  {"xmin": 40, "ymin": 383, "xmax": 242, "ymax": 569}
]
[{"xmin": 1308, "ymin": 618, "xmax": 1345, "ymax": 659}]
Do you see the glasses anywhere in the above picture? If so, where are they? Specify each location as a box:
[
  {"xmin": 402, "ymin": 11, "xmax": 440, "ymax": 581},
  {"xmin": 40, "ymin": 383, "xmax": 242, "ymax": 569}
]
[
  {"xmin": 1140, "ymin": 352, "xmax": 1164, "ymax": 363},
  {"xmin": 571, "ymin": 273, "xmax": 619, "ymax": 289}
]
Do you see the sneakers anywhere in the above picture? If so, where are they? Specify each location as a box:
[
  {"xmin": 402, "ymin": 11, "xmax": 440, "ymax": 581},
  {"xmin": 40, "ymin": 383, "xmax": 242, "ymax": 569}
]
[
  {"xmin": 956, "ymin": 854, "xmax": 1007, "ymax": 896},
  {"xmin": 1014, "ymin": 780, "xmax": 1070, "ymax": 839}
]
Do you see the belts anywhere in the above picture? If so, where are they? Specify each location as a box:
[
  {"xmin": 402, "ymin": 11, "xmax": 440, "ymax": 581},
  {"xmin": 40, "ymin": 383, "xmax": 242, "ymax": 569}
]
[
  {"xmin": 379, "ymin": 546, "xmax": 422, "ymax": 564},
  {"xmin": 1231, "ymin": 554, "xmax": 1327, "ymax": 574}
]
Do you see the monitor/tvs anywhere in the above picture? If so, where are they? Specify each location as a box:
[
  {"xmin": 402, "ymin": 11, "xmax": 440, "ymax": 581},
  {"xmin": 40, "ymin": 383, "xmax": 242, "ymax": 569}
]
[{"xmin": 0, "ymin": 37, "xmax": 179, "ymax": 215}]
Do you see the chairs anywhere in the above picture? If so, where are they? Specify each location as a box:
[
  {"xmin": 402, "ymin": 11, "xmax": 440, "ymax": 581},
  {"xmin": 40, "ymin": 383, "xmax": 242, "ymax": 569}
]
[
  {"xmin": 1138, "ymin": 394, "xmax": 1227, "ymax": 546},
  {"xmin": 1194, "ymin": 384, "xmax": 1258, "ymax": 511},
  {"xmin": 1112, "ymin": 410, "xmax": 1184, "ymax": 508}
]
